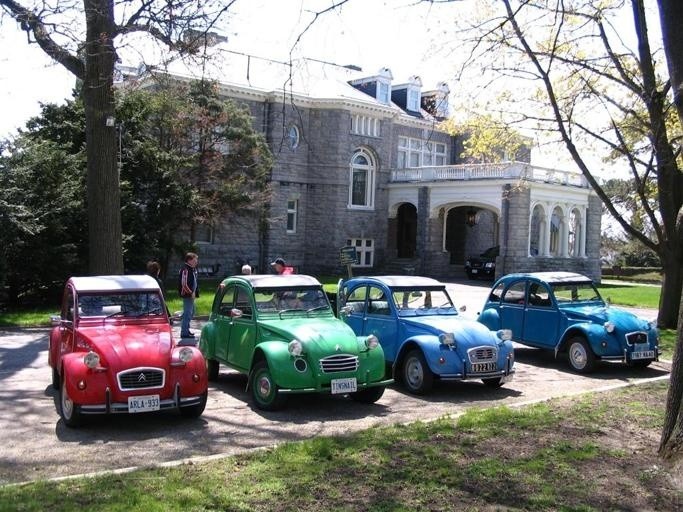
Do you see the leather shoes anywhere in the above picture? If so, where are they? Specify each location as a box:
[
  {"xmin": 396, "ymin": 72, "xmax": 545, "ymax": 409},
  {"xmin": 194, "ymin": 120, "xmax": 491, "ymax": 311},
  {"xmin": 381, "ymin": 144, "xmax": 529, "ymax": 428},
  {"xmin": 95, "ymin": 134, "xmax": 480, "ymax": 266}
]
[{"xmin": 181, "ymin": 331, "xmax": 195, "ymax": 338}]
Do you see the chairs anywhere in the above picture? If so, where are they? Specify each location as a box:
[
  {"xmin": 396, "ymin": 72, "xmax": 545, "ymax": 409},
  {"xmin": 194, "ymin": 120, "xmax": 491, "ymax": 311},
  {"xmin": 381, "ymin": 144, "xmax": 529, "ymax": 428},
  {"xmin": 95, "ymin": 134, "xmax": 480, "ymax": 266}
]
[
  {"xmin": 347, "ymin": 297, "xmax": 389, "ymax": 316},
  {"xmin": 83, "ymin": 295, "xmax": 142, "ymax": 318}
]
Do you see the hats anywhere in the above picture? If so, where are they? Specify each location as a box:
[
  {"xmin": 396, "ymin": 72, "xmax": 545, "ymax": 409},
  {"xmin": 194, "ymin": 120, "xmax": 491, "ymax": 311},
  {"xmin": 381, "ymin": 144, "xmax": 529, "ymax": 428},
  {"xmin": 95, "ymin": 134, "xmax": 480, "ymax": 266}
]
[{"xmin": 270, "ymin": 258, "xmax": 285, "ymax": 267}]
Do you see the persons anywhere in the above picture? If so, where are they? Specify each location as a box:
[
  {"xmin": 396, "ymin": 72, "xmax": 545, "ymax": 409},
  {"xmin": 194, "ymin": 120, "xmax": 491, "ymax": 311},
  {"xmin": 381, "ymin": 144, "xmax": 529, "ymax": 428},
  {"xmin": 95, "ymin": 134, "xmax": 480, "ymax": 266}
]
[
  {"xmin": 179, "ymin": 252, "xmax": 200, "ymax": 338},
  {"xmin": 274, "ymin": 258, "xmax": 291, "ymax": 274},
  {"xmin": 237, "ymin": 264, "xmax": 252, "ymax": 302},
  {"xmin": 147, "ymin": 261, "xmax": 165, "ymax": 300}
]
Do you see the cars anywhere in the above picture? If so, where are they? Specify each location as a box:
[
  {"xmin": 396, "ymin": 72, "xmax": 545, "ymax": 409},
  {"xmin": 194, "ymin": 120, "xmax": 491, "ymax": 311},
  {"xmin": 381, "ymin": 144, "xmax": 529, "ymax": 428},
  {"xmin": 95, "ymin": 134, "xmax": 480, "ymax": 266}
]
[
  {"xmin": 334, "ymin": 273, "xmax": 514, "ymax": 391},
  {"xmin": 477, "ymin": 271, "xmax": 660, "ymax": 370},
  {"xmin": 48, "ymin": 275, "xmax": 209, "ymax": 424},
  {"xmin": 199, "ymin": 274, "xmax": 388, "ymax": 408},
  {"xmin": 464, "ymin": 245, "xmax": 499, "ymax": 279}
]
